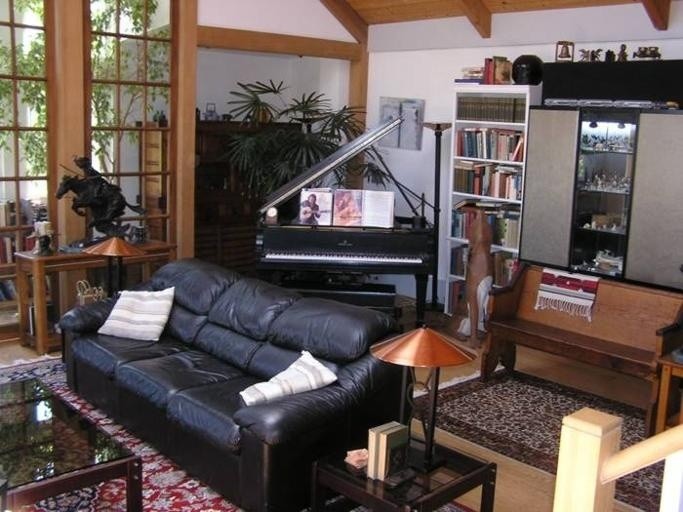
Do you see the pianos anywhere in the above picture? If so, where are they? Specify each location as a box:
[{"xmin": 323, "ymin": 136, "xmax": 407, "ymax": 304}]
[{"xmin": 253, "ymin": 117, "xmax": 438, "ymax": 327}]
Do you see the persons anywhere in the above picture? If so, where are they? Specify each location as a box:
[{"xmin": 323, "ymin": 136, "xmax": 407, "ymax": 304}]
[
  {"xmin": 301, "ymin": 194, "xmax": 320, "ymax": 225},
  {"xmin": 77, "ymin": 156, "xmax": 109, "ymax": 198},
  {"xmin": 336, "ymin": 191, "xmax": 360, "ymax": 217}
]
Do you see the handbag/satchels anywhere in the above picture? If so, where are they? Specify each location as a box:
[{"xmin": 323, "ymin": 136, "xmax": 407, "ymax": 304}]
[{"xmin": 76, "ymin": 280, "xmax": 109, "ymax": 306}]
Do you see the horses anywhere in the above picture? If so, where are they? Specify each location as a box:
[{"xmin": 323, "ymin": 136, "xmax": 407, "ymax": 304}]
[{"xmin": 55, "ymin": 173, "xmax": 148, "ymax": 243}]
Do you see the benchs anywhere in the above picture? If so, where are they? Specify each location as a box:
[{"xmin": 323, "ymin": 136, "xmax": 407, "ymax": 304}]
[
  {"xmin": 477, "ymin": 261, "xmax": 680, "ymax": 436},
  {"xmin": 281, "ymin": 280, "xmax": 395, "ymax": 317}
]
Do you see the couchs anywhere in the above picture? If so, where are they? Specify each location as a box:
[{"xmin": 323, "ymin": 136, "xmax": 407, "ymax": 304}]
[{"xmin": 57, "ymin": 258, "xmax": 414, "ymax": 511}]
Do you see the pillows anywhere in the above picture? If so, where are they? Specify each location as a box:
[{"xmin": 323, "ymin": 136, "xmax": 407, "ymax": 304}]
[
  {"xmin": 94, "ymin": 282, "xmax": 175, "ymax": 345},
  {"xmin": 237, "ymin": 350, "xmax": 338, "ymax": 409}
]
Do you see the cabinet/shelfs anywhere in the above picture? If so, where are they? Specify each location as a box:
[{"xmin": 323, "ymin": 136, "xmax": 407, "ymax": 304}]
[
  {"xmin": 140, "ymin": 115, "xmax": 305, "ymax": 282},
  {"xmin": 564, "ymin": 110, "xmax": 638, "ymax": 290},
  {"xmin": 443, "ymin": 79, "xmax": 545, "ymax": 334}
]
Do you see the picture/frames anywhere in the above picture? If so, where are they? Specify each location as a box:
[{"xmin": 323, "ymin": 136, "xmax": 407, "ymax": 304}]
[{"xmin": 553, "ymin": 40, "xmax": 575, "ymax": 62}]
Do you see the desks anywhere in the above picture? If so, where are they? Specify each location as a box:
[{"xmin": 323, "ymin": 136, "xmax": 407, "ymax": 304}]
[
  {"xmin": 11, "ymin": 238, "xmax": 176, "ymax": 356},
  {"xmin": 654, "ymin": 353, "xmax": 680, "ymax": 436}
]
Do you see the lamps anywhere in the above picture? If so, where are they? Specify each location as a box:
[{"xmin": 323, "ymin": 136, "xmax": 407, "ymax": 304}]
[
  {"xmin": 81, "ymin": 237, "xmax": 144, "ymax": 298},
  {"xmin": 366, "ymin": 329, "xmax": 480, "ymax": 475}
]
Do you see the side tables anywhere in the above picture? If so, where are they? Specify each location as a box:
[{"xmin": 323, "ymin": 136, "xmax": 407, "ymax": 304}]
[{"xmin": 311, "ymin": 432, "xmax": 497, "ymax": 512}]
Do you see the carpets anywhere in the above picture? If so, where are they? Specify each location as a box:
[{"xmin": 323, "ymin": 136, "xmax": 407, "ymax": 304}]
[
  {"xmin": 403, "ymin": 367, "xmax": 670, "ymax": 510},
  {"xmin": 1, "ymin": 351, "xmax": 481, "ymax": 511}
]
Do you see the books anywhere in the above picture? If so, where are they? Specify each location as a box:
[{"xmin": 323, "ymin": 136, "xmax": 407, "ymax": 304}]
[
  {"xmin": 448, "ymin": 97, "xmax": 526, "ymax": 315},
  {"xmin": 367, "ymin": 421, "xmax": 409, "ymax": 482},
  {"xmin": 0, "ymin": 198, "xmax": 51, "ymax": 302}
]
